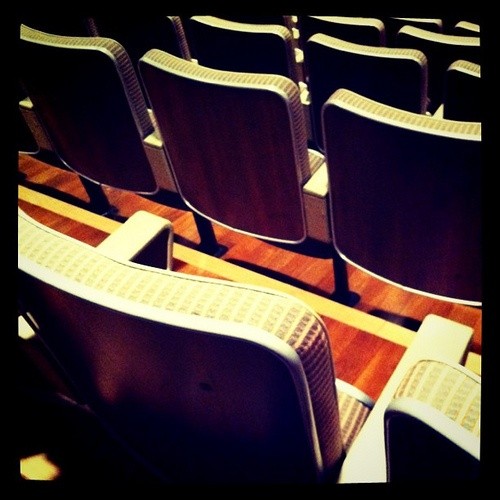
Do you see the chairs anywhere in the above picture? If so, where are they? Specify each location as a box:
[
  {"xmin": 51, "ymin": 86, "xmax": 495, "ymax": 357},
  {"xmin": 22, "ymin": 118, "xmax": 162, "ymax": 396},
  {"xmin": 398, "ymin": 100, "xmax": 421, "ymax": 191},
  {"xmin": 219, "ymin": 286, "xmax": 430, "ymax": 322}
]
[{"xmin": 17, "ymin": 16, "xmax": 482, "ymax": 488}]
